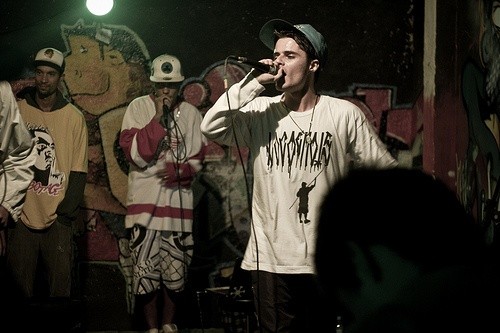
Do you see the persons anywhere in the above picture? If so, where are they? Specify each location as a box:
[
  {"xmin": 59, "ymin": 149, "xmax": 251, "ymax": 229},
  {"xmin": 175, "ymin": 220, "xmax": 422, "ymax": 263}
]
[
  {"xmin": 201, "ymin": 20, "xmax": 398, "ymax": 333},
  {"xmin": 0, "ymin": 81, "xmax": 38, "ymax": 230},
  {"xmin": 5, "ymin": 45, "xmax": 87, "ymax": 295},
  {"xmin": 312, "ymin": 165, "xmax": 500, "ymax": 333},
  {"xmin": 120, "ymin": 54, "xmax": 207, "ymax": 333}
]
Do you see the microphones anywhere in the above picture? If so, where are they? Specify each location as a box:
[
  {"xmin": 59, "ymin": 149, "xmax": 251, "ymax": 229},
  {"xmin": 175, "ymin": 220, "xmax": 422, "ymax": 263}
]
[
  {"xmin": 233, "ymin": 56, "xmax": 279, "ymax": 76},
  {"xmin": 163, "ymin": 98, "xmax": 171, "ymax": 123}
]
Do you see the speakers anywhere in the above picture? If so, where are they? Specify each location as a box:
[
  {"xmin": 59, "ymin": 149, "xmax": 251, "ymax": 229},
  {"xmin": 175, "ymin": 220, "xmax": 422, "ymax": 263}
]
[{"xmin": 77, "ymin": 260, "xmax": 141, "ymax": 333}]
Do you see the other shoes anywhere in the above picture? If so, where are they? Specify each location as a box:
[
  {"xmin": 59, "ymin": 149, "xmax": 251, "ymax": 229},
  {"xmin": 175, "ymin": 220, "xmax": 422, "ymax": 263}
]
[
  {"xmin": 148, "ymin": 326, "xmax": 160, "ymax": 333},
  {"xmin": 162, "ymin": 323, "xmax": 179, "ymax": 333}
]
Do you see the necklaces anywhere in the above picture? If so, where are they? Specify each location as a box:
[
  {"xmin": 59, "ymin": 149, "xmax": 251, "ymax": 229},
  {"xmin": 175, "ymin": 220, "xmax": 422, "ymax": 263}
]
[
  {"xmin": 163, "ymin": 104, "xmax": 180, "ymax": 130},
  {"xmin": 279, "ymin": 94, "xmax": 319, "ymax": 144}
]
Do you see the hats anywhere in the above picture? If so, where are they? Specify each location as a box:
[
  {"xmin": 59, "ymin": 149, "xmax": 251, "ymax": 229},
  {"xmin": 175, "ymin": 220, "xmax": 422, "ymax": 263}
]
[
  {"xmin": 30, "ymin": 48, "xmax": 65, "ymax": 74},
  {"xmin": 259, "ymin": 19, "xmax": 326, "ymax": 62},
  {"xmin": 150, "ymin": 54, "xmax": 184, "ymax": 83}
]
